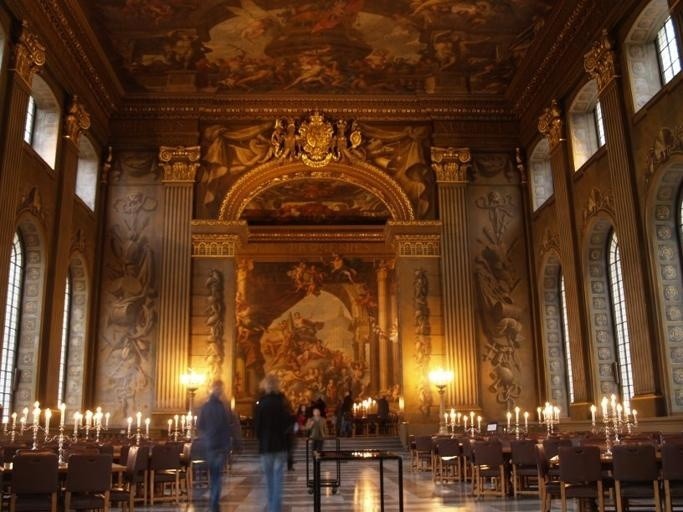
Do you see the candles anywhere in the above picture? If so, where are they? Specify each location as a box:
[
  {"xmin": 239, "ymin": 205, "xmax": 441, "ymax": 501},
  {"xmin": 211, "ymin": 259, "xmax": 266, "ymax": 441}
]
[
  {"xmin": 441, "ymin": 394, "xmax": 641, "ymax": 431},
  {"xmin": 1, "ymin": 399, "xmax": 196, "ymax": 447},
  {"xmin": 352, "ymin": 396, "xmax": 378, "ymax": 416}
]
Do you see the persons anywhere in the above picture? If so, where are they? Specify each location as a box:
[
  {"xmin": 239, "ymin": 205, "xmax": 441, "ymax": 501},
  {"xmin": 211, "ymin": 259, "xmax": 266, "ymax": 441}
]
[
  {"xmin": 194, "ymin": 381, "xmax": 245, "ymax": 512},
  {"xmin": 172, "ymin": 4, "xmax": 506, "ymax": 93},
  {"xmin": 231, "ymin": 249, "xmax": 389, "ymax": 448},
  {"xmin": 248, "ymin": 376, "xmax": 295, "ymax": 512}
]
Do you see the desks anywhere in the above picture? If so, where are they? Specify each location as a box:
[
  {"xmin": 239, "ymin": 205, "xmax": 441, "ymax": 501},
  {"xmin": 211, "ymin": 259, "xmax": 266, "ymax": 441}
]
[{"xmin": 311, "ymin": 451, "xmax": 403, "ymax": 512}]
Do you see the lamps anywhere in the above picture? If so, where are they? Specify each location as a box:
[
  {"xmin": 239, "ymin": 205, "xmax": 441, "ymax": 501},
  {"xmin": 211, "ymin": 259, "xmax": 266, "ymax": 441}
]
[
  {"xmin": 178, "ymin": 369, "xmax": 204, "ymax": 409},
  {"xmin": 428, "ymin": 368, "xmax": 453, "ymax": 410}
]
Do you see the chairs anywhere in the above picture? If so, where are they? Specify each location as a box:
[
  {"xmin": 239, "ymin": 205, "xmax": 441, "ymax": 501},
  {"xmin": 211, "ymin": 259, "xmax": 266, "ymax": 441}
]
[
  {"xmin": 408, "ymin": 429, "xmax": 681, "ymax": 510},
  {"xmin": 7, "ymin": 441, "xmax": 208, "ymax": 511}
]
